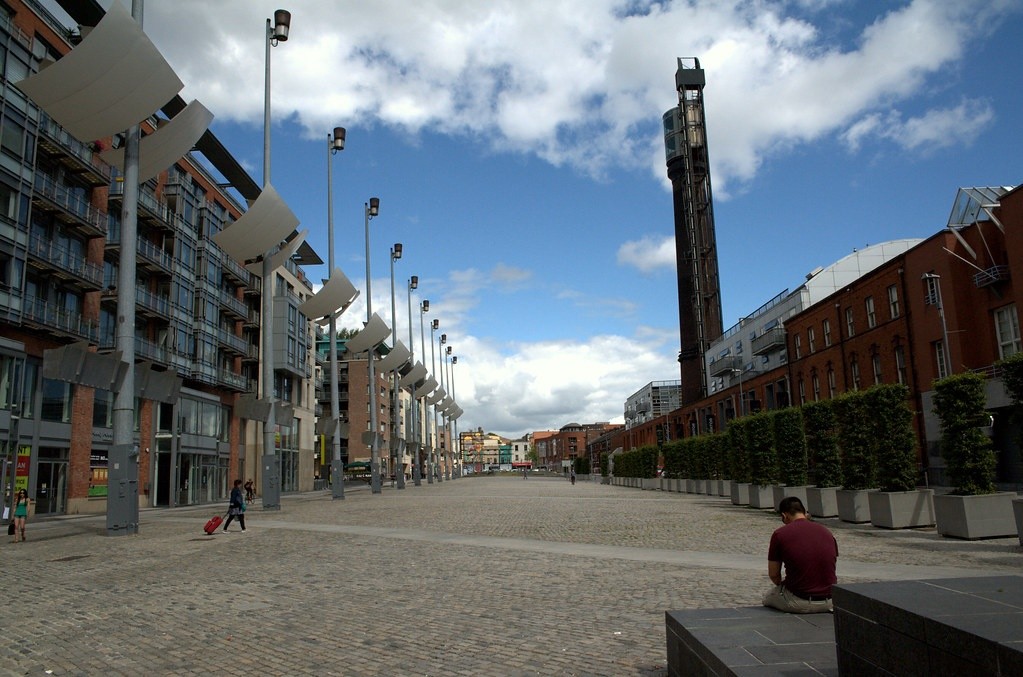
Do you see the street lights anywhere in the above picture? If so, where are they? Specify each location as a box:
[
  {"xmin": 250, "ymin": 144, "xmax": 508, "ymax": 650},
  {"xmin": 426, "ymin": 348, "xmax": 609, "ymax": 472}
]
[
  {"xmin": 921, "ymin": 272, "xmax": 954, "ymax": 376},
  {"xmin": 732, "ymin": 368, "xmax": 745, "ymax": 415},
  {"xmin": 261, "ymin": 7, "xmax": 463, "ymax": 509}
]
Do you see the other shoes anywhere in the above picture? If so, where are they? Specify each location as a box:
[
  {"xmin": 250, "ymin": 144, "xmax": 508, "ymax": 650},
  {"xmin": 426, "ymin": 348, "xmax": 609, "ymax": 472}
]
[
  {"xmin": 223, "ymin": 529, "xmax": 231, "ymax": 534},
  {"xmin": 242, "ymin": 528, "xmax": 250, "ymax": 533}
]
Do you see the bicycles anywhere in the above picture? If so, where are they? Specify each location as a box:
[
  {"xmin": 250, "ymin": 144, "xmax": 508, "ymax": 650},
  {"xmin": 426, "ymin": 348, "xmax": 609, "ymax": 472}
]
[{"xmin": 243, "ymin": 491, "xmax": 257, "ymax": 505}]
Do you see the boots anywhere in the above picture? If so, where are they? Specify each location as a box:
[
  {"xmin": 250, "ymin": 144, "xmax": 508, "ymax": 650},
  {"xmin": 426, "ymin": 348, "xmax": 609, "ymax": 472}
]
[
  {"xmin": 20, "ymin": 528, "xmax": 26, "ymax": 542},
  {"xmin": 14, "ymin": 528, "xmax": 19, "ymax": 543}
]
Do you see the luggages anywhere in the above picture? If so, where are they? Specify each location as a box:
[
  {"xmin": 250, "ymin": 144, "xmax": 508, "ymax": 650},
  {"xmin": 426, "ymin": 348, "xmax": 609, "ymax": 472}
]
[{"xmin": 203, "ymin": 509, "xmax": 230, "ymax": 534}]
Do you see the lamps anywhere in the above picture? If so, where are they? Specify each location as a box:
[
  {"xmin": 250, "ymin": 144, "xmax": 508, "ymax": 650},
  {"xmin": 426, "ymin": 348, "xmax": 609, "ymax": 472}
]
[
  {"xmin": 423, "ymin": 300, "xmax": 429, "ymax": 311},
  {"xmin": 448, "ymin": 346, "xmax": 451, "ymax": 354},
  {"xmin": 442, "ymin": 335, "xmax": 446, "ymax": 343},
  {"xmin": 333, "ymin": 127, "xmax": 346, "ymax": 150},
  {"xmin": 394, "ymin": 243, "xmax": 402, "ymax": 258},
  {"xmin": 274, "ymin": 10, "xmax": 290, "ymax": 41},
  {"xmin": 453, "ymin": 357, "xmax": 457, "ymax": 364},
  {"xmin": 411, "ymin": 276, "xmax": 418, "ymax": 288},
  {"xmin": 370, "ymin": 198, "xmax": 379, "ymax": 215},
  {"xmin": 434, "ymin": 319, "xmax": 438, "ymax": 329}
]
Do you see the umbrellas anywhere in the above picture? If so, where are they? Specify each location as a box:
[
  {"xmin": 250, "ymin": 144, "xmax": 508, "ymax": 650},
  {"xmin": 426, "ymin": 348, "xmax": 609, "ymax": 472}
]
[{"xmin": 344, "ymin": 461, "xmax": 371, "ymax": 473}]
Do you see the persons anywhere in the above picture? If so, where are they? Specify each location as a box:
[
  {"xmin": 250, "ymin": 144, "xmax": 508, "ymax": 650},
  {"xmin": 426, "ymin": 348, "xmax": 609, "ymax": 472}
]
[
  {"xmin": 245, "ymin": 479, "xmax": 254, "ymax": 503},
  {"xmin": 761, "ymin": 496, "xmax": 839, "ymax": 613},
  {"xmin": 222, "ymin": 479, "xmax": 249, "ymax": 533},
  {"xmin": 571, "ymin": 470, "xmax": 576, "ymax": 485},
  {"xmin": 523, "ymin": 468, "xmax": 528, "ymax": 479},
  {"xmin": 11, "ymin": 489, "xmax": 30, "ymax": 543}
]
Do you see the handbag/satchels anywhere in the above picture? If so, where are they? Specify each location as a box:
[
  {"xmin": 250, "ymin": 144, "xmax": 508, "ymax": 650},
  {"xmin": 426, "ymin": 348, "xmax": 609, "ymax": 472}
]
[
  {"xmin": 7, "ymin": 520, "xmax": 15, "ymax": 535},
  {"xmin": 245, "ymin": 484, "xmax": 250, "ymax": 487}
]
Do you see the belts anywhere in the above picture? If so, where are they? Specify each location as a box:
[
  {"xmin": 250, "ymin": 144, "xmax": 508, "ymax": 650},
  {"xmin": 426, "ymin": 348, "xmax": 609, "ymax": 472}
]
[{"xmin": 785, "ymin": 585, "xmax": 833, "ymax": 601}]
